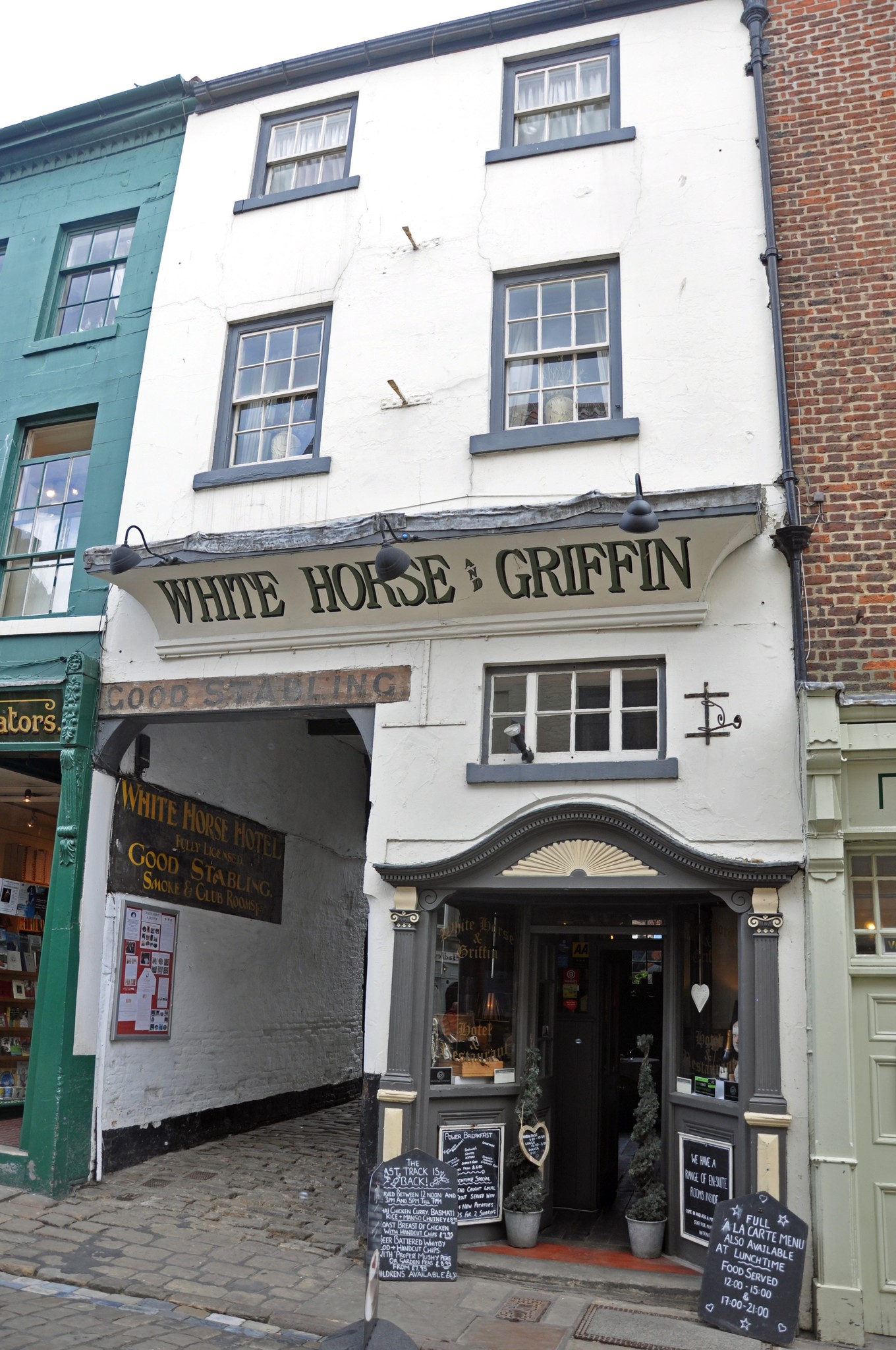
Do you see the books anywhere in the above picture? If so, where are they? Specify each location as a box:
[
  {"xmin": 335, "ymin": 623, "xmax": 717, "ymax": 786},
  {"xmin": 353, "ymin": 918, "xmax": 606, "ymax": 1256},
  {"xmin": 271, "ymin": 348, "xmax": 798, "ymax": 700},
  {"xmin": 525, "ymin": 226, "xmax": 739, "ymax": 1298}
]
[{"xmin": 0, "ymin": 926, "xmax": 42, "ymax": 1101}]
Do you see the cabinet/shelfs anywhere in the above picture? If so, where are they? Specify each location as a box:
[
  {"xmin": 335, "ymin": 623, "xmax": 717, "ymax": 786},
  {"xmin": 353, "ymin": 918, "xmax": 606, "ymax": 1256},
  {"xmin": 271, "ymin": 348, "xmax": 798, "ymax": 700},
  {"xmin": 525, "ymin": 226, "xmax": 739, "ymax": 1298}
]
[{"xmin": 0, "ymin": 912, "xmax": 45, "ymax": 1121}]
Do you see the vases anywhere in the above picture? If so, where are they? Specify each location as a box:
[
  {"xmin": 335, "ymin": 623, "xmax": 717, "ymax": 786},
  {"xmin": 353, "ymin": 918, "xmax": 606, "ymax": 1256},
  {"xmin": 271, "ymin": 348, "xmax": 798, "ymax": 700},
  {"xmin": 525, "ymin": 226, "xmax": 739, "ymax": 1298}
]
[
  {"xmin": 544, "ymin": 391, "xmax": 573, "ymax": 424},
  {"xmin": 271, "ymin": 430, "xmax": 302, "ymax": 459}
]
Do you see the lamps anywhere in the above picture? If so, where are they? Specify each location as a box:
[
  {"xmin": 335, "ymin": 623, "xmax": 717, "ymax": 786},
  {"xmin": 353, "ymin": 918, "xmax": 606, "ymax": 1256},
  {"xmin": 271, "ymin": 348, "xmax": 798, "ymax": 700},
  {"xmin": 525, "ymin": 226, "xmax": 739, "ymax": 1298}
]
[
  {"xmin": 618, "ymin": 473, "xmax": 659, "ymax": 534},
  {"xmin": 23, "ymin": 788, "xmax": 31, "ymax": 802},
  {"xmin": 503, "ymin": 722, "xmax": 535, "ymax": 764},
  {"xmin": 27, "ymin": 811, "xmax": 37, "ymax": 828},
  {"xmin": 110, "ymin": 525, "xmax": 173, "ymax": 576},
  {"xmin": 375, "ymin": 518, "xmax": 412, "ymax": 582}
]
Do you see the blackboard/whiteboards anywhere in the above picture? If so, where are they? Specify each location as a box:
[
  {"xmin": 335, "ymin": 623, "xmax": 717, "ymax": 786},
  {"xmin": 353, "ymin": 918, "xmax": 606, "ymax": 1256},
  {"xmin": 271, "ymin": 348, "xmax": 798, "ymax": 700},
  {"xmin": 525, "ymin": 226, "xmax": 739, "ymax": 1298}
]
[
  {"xmin": 698, "ymin": 1191, "xmax": 808, "ymax": 1346},
  {"xmin": 366, "ymin": 1148, "xmax": 458, "ymax": 1281},
  {"xmin": 438, "ymin": 1123, "xmax": 506, "ymax": 1226},
  {"xmin": 518, "ymin": 1123, "xmax": 549, "ymax": 1166},
  {"xmin": 678, "ymin": 1132, "xmax": 734, "ymax": 1247}
]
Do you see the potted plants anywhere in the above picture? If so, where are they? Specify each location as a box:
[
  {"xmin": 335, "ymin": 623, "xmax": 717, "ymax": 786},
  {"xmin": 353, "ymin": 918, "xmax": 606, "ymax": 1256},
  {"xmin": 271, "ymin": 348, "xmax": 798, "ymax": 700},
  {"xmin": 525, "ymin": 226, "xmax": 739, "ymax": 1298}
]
[
  {"xmin": 625, "ymin": 1033, "xmax": 669, "ymax": 1258},
  {"xmin": 501, "ymin": 1047, "xmax": 546, "ymax": 1249}
]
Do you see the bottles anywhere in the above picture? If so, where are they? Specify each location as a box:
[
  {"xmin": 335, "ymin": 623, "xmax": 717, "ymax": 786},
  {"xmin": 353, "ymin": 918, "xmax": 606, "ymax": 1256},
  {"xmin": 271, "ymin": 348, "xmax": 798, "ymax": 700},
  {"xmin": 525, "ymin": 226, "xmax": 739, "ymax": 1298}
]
[{"xmin": 719, "ymin": 1029, "xmax": 734, "ymax": 1081}]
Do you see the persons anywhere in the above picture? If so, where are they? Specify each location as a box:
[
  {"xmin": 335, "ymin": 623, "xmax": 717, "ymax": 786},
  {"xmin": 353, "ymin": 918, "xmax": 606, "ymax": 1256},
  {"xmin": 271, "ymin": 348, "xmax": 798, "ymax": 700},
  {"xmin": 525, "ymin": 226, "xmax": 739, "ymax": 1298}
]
[
  {"xmin": 26, "ymin": 885, "xmax": 37, "ymax": 906},
  {"xmin": 126, "ymin": 943, "xmax": 135, "ymax": 953},
  {"xmin": 732, "ymin": 1021, "xmax": 739, "ymax": 1083},
  {"xmin": 34, "ymin": 908, "xmax": 41, "ymax": 919},
  {"xmin": 142, "ymin": 955, "xmax": 149, "ymax": 964}
]
